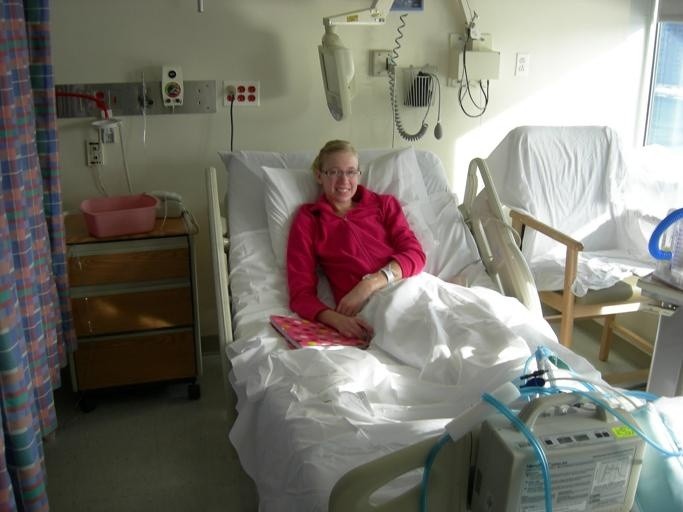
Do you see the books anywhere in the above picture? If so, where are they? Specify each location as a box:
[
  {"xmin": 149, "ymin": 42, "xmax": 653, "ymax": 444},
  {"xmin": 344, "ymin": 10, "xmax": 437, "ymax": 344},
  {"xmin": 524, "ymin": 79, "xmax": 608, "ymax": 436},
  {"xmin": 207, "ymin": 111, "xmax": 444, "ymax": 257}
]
[{"xmin": 269, "ymin": 315, "xmax": 368, "ymax": 351}]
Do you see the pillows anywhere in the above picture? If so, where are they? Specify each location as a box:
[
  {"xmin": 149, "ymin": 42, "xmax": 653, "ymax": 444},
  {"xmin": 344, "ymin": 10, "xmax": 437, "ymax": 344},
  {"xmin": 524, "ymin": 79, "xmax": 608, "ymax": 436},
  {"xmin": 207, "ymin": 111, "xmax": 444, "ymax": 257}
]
[{"xmin": 262, "ymin": 148, "xmax": 442, "ymax": 270}]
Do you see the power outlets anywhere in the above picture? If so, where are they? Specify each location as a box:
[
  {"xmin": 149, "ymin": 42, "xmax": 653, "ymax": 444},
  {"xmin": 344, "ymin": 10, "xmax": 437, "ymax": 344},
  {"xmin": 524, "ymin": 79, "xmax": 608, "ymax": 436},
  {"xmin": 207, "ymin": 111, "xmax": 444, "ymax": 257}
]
[
  {"xmin": 220, "ymin": 80, "xmax": 261, "ymax": 104},
  {"xmin": 86, "ymin": 138, "xmax": 105, "ymax": 166}
]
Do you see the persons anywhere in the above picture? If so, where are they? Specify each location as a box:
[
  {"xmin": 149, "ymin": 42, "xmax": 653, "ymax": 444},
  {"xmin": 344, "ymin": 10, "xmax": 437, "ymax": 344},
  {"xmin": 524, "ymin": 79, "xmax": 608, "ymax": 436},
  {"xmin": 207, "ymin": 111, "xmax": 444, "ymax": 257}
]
[{"xmin": 285, "ymin": 140, "xmax": 426, "ymax": 342}]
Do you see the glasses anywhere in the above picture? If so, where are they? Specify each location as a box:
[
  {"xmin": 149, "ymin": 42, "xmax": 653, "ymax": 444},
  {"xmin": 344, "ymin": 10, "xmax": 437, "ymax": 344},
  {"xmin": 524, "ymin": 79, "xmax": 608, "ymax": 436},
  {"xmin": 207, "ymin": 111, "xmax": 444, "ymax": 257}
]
[{"xmin": 321, "ymin": 167, "xmax": 362, "ymax": 178}]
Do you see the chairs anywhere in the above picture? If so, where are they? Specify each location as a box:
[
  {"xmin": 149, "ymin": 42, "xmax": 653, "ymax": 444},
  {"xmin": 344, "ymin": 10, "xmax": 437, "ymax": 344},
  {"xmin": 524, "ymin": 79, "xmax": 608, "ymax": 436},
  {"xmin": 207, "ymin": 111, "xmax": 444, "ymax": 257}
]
[{"xmin": 503, "ymin": 127, "xmax": 668, "ymax": 381}]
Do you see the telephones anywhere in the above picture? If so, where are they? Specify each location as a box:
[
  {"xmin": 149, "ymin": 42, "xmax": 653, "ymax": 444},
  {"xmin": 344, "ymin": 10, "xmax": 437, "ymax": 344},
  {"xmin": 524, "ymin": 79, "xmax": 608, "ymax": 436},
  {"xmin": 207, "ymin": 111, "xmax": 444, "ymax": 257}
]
[{"xmin": 150, "ymin": 191, "xmax": 183, "ymax": 218}]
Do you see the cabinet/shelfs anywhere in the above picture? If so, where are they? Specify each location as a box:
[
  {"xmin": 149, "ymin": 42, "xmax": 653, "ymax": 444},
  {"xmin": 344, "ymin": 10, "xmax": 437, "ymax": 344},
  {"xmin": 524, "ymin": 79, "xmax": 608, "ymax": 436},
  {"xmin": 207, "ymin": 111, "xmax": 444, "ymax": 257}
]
[{"xmin": 60, "ymin": 207, "xmax": 203, "ymax": 414}]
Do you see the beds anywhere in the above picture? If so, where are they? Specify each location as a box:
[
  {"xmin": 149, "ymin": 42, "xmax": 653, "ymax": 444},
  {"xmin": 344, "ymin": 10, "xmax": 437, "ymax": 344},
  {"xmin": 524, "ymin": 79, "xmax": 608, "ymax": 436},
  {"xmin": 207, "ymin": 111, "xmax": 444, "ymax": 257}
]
[{"xmin": 207, "ymin": 145, "xmax": 679, "ymax": 511}]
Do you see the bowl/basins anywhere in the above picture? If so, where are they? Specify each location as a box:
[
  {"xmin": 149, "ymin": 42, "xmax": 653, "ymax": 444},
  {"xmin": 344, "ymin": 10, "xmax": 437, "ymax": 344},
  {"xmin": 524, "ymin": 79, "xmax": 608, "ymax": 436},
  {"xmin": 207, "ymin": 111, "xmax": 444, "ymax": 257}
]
[{"xmin": 80, "ymin": 192, "xmax": 160, "ymax": 239}]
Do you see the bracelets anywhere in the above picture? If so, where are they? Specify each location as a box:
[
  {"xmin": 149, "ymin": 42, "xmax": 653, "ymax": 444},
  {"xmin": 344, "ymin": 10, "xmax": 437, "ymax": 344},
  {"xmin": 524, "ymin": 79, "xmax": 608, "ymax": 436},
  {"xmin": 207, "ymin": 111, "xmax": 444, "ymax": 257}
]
[{"xmin": 379, "ymin": 267, "xmax": 395, "ymax": 285}]
